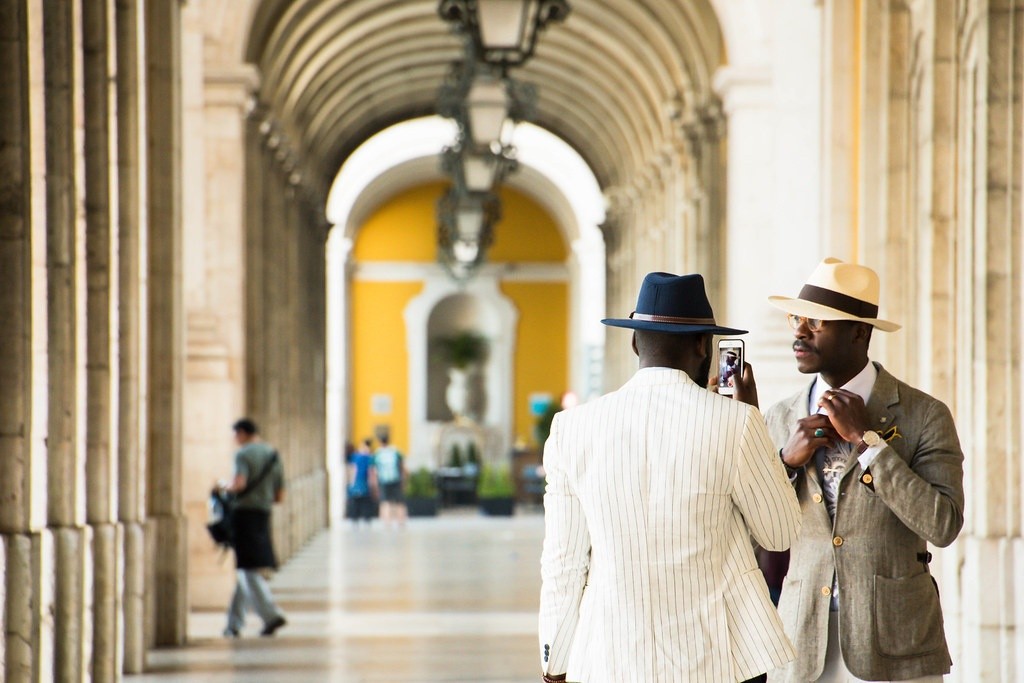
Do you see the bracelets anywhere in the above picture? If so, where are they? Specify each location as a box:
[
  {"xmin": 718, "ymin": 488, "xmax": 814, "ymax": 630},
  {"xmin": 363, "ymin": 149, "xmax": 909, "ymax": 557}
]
[{"xmin": 779, "ymin": 448, "xmax": 800, "ymax": 474}]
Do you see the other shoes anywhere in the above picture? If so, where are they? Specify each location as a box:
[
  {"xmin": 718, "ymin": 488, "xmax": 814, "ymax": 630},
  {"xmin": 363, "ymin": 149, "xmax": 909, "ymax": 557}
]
[
  {"xmin": 261, "ymin": 618, "xmax": 286, "ymax": 635},
  {"xmin": 222, "ymin": 628, "xmax": 238, "ymax": 637}
]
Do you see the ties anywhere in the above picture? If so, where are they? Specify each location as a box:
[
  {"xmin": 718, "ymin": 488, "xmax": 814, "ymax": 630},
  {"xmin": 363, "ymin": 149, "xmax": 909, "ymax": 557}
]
[{"xmin": 822, "ymin": 429, "xmax": 851, "ymax": 597}]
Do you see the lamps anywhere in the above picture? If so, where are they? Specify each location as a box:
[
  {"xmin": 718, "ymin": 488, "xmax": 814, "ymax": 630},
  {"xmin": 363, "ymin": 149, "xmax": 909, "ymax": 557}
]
[{"xmin": 435, "ymin": 0, "xmax": 571, "ymax": 283}]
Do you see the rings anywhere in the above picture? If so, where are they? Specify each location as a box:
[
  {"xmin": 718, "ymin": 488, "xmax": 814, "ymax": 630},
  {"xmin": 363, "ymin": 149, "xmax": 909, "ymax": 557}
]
[
  {"xmin": 827, "ymin": 395, "xmax": 836, "ymax": 400},
  {"xmin": 814, "ymin": 428, "xmax": 824, "ymax": 437}
]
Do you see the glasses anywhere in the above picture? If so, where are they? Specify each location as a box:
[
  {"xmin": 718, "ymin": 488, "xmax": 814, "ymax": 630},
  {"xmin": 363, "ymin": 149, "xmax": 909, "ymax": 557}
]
[{"xmin": 788, "ymin": 313, "xmax": 857, "ymax": 332}]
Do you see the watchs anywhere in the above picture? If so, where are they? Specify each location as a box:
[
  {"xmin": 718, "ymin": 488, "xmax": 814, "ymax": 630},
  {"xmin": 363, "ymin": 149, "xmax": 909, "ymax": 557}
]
[{"xmin": 857, "ymin": 429, "xmax": 882, "ymax": 454}]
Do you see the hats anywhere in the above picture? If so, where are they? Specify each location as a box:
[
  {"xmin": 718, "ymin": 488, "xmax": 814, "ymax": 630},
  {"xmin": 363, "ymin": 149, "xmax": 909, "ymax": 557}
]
[
  {"xmin": 600, "ymin": 272, "xmax": 749, "ymax": 335},
  {"xmin": 723, "ymin": 350, "xmax": 740, "ymax": 359},
  {"xmin": 768, "ymin": 257, "xmax": 902, "ymax": 332}
]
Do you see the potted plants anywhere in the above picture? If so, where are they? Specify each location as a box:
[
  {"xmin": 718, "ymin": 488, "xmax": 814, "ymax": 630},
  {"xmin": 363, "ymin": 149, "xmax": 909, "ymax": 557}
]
[
  {"xmin": 436, "ymin": 333, "xmax": 488, "ymax": 415},
  {"xmin": 404, "ymin": 464, "xmax": 441, "ymax": 517},
  {"xmin": 477, "ymin": 465, "xmax": 517, "ymax": 515}
]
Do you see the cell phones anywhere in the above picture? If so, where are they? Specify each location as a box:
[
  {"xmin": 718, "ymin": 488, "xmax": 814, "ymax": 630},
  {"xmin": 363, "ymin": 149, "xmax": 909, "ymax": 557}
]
[{"xmin": 716, "ymin": 338, "xmax": 744, "ymax": 397}]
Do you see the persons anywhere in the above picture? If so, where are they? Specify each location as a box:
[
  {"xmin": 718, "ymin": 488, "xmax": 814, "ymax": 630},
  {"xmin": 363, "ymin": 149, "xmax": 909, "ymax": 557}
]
[
  {"xmin": 718, "ymin": 350, "xmax": 741, "ymax": 387},
  {"xmin": 344, "ymin": 432, "xmax": 401, "ymax": 524},
  {"xmin": 537, "ymin": 272, "xmax": 802, "ymax": 683},
  {"xmin": 213, "ymin": 419, "xmax": 287, "ymax": 638},
  {"xmin": 761, "ymin": 257, "xmax": 965, "ymax": 683}
]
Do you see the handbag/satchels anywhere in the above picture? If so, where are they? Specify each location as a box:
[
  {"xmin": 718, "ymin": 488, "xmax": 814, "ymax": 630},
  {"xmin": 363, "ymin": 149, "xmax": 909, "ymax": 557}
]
[{"xmin": 207, "ymin": 491, "xmax": 242, "ymax": 543}]
